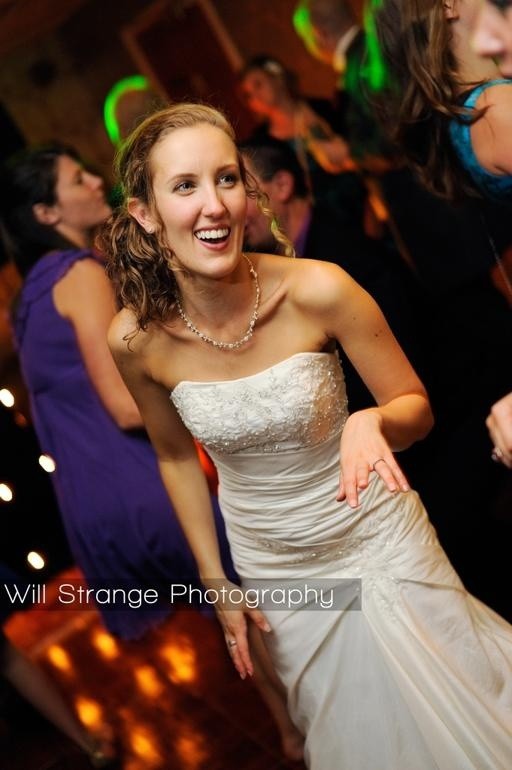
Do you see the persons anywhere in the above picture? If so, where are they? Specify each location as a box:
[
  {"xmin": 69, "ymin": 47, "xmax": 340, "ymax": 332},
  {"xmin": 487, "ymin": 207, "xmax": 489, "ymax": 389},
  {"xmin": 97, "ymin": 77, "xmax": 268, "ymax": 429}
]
[
  {"xmin": 229, "ymin": 1, "xmax": 512, "ymax": 470},
  {"xmin": 94, "ymin": 100, "xmax": 511, "ymax": 770},
  {"xmin": 1, "ymin": 139, "xmax": 232, "ymax": 652}
]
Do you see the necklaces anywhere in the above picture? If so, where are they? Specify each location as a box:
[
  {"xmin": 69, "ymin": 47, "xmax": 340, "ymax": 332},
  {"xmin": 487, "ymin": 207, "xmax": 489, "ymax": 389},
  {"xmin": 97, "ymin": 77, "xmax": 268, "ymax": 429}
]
[{"xmin": 172, "ymin": 251, "xmax": 261, "ymax": 350}]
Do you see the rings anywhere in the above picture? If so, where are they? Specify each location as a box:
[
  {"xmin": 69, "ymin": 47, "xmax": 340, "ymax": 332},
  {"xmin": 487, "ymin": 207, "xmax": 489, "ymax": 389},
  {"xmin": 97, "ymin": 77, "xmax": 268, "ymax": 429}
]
[{"xmin": 227, "ymin": 640, "xmax": 236, "ymax": 646}]
